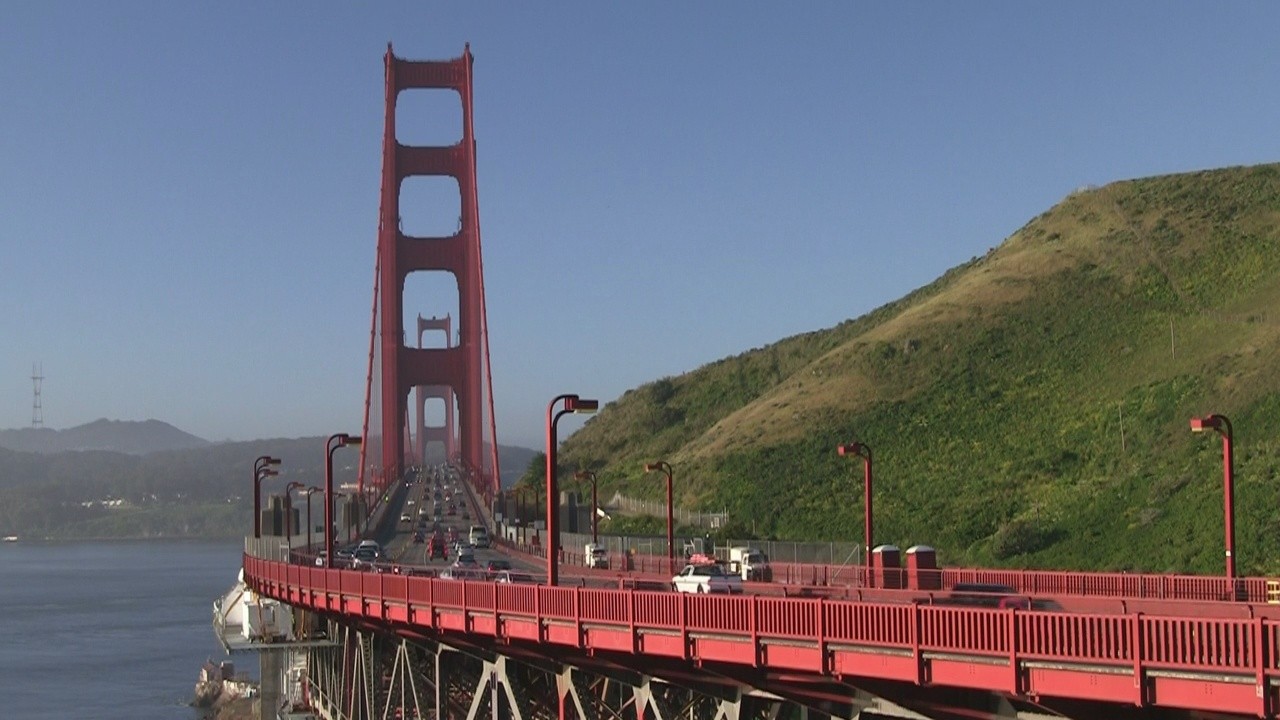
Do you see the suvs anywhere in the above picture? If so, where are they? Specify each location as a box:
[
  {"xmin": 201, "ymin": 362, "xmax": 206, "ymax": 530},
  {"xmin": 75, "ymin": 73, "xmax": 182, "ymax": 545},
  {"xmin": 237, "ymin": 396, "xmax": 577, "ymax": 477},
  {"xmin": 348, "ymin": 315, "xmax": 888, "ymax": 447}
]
[
  {"xmin": 488, "ymin": 560, "xmax": 511, "ymax": 574},
  {"xmin": 949, "ymin": 583, "xmax": 1054, "ymax": 613}
]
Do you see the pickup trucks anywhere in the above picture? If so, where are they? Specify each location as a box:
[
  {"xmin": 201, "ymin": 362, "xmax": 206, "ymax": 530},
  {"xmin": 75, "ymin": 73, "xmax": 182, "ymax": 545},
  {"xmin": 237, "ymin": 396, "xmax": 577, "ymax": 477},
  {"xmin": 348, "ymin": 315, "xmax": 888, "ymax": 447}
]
[{"xmin": 668, "ymin": 564, "xmax": 745, "ymax": 596}]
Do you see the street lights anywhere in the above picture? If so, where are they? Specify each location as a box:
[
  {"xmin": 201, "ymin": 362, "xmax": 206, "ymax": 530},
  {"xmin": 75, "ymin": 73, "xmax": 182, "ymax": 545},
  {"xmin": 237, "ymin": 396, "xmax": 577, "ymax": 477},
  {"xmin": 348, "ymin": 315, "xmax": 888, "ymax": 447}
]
[
  {"xmin": 324, "ymin": 432, "xmax": 362, "ymax": 569},
  {"xmin": 645, "ymin": 461, "xmax": 675, "ymax": 579},
  {"xmin": 838, "ymin": 443, "xmax": 874, "ymax": 588},
  {"xmin": 1189, "ymin": 413, "xmax": 1237, "ymax": 601},
  {"xmin": 285, "ymin": 482, "xmax": 305, "ymax": 548},
  {"xmin": 332, "ymin": 491, "xmax": 347, "ymax": 545},
  {"xmin": 574, "ymin": 472, "xmax": 598, "ymax": 544},
  {"xmin": 545, "ymin": 393, "xmax": 599, "ymax": 587},
  {"xmin": 304, "ymin": 485, "xmax": 324, "ymax": 554},
  {"xmin": 252, "ymin": 455, "xmax": 284, "ymax": 541}
]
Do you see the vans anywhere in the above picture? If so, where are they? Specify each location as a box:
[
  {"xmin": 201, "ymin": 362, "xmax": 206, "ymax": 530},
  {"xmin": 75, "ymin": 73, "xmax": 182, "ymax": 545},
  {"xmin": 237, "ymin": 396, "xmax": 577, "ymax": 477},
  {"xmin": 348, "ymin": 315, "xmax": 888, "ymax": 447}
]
[{"xmin": 468, "ymin": 524, "xmax": 488, "ymax": 545}]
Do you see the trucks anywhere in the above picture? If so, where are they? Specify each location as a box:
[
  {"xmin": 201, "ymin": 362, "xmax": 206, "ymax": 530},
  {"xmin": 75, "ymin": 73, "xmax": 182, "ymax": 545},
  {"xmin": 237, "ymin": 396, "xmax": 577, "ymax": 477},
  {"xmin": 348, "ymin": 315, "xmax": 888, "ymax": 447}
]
[
  {"xmin": 584, "ymin": 543, "xmax": 610, "ymax": 569},
  {"xmin": 726, "ymin": 547, "xmax": 772, "ymax": 582}
]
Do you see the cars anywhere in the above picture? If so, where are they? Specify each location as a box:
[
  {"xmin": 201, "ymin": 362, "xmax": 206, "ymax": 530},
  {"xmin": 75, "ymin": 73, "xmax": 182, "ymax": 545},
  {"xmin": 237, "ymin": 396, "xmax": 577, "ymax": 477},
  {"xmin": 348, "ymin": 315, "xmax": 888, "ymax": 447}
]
[
  {"xmin": 400, "ymin": 512, "xmax": 411, "ymax": 522},
  {"xmin": 407, "ymin": 498, "xmax": 415, "ymax": 506},
  {"xmin": 475, "ymin": 535, "xmax": 490, "ymax": 550},
  {"xmin": 493, "ymin": 570, "xmax": 536, "ymax": 586},
  {"xmin": 413, "ymin": 460, "xmax": 492, "ymax": 582},
  {"xmin": 313, "ymin": 541, "xmax": 386, "ymax": 572}
]
